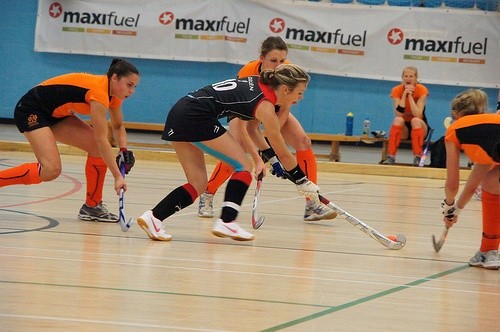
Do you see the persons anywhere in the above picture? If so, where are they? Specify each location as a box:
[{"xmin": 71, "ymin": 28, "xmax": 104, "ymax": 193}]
[
  {"xmin": 136, "ymin": 35, "xmax": 338, "ymax": 241},
  {"xmin": 440, "ymin": 88, "xmax": 500, "ymax": 270},
  {"xmin": 381, "ymin": 66, "xmax": 430, "ymax": 167},
  {"xmin": 0, "ymin": 58, "xmax": 140, "ymax": 223}
]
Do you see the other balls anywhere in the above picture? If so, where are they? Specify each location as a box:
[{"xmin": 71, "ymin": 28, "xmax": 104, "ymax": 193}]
[{"xmin": 387, "ymin": 235, "xmax": 398, "ymax": 243}]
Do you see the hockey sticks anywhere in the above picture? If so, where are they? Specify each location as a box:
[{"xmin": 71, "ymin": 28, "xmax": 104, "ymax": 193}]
[
  {"xmin": 269, "ymin": 167, "xmax": 407, "ymax": 250},
  {"xmin": 119, "ymin": 161, "xmax": 134, "ymax": 233},
  {"xmin": 433, "ymin": 219, "xmax": 452, "ymax": 253},
  {"xmin": 252, "ymin": 171, "xmax": 266, "ymax": 229},
  {"xmin": 418, "ymin": 128, "xmax": 434, "ymax": 167}
]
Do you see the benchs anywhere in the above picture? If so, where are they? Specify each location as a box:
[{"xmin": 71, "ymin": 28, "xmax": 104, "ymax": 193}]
[{"xmin": 84, "ymin": 120, "xmax": 428, "ymax": 165}]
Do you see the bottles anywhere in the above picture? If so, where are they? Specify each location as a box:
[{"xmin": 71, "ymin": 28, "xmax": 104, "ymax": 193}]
[
  {"xmin": 363, "ymin": 118, "xmax": 370, "ymax": 137},
  {"xmin": 345, "ymin": 112, "xmax": 354, "ymax": 136}
]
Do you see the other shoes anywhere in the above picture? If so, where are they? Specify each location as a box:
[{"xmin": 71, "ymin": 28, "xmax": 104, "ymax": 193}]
[
  {"xmin": 381, "ymin": 156, "xmax": 395, "ymax": 164},
  {"xmin": 414, "ymin": 156, "xmax": 421, "ymax": 166}
]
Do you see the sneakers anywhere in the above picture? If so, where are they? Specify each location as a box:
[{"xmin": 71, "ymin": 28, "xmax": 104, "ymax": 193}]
[
  {"xmin": 197, "ymin": 192, "xmax": 214, "ymax": 217},
  {"xmin": 212, "ymin": 218, "xmax": 256, "ymax": 241},
  {"xmin": 304, "ymin": 203, "xmax": 337, "ymax": 220},
  {"xmin": 77, "ymin": 201, "xmax": 120, "ymax": 222},
  {"xmin": 468, "ymin": 248, "xmax": 500, "ymax": 269},
  {"xmin": 137, "ymin": 210, "xmax": 172, "ymax": 240}
]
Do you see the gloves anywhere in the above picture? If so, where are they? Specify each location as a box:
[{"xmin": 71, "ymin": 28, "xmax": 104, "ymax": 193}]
[
  {"xmin": 116, "ymin": 148, "xmax": 135, "ymax": 174},
  {"xmin": 296, "ymin": 176, "xmax": 320, "ymax": 198},
  {"xmin": 269, "ymin": 156, "xmax": 287, "ymax": 179},
  {"xmin": 441, "ymin": 199, "xmax": 456, "ymax": 218}
]
